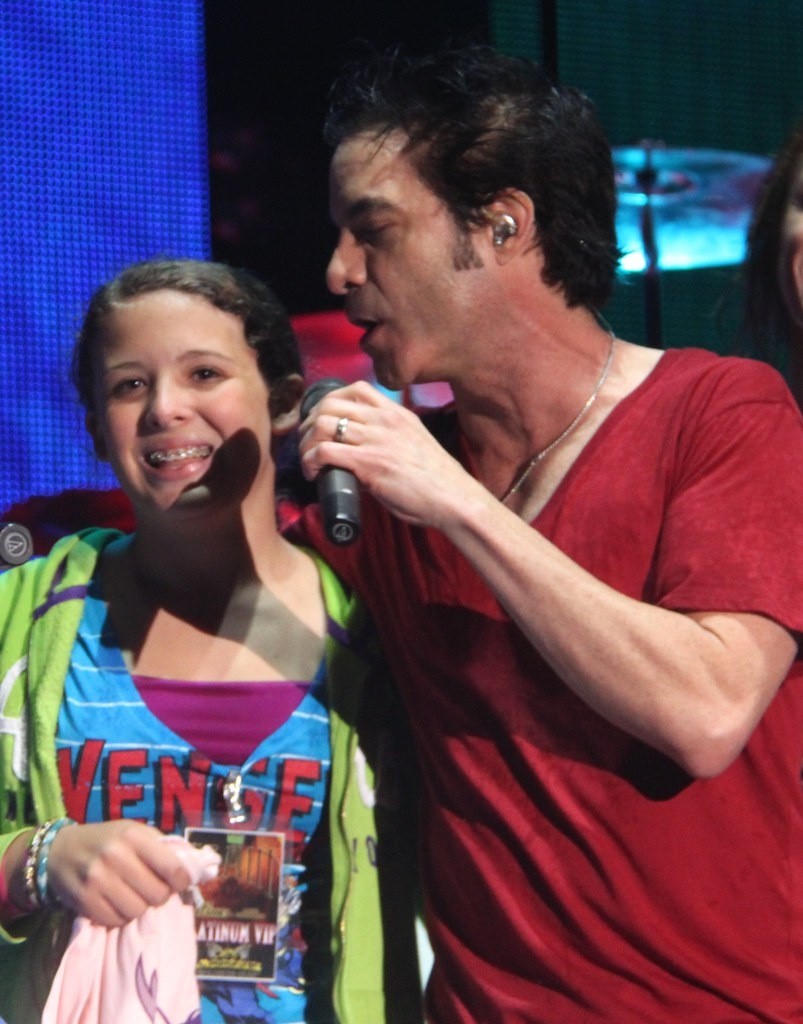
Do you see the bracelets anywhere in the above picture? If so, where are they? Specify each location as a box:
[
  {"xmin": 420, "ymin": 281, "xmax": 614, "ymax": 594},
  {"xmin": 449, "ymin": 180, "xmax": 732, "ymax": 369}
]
[{"xmin": 22, "ymin": 816, "xmax": 75, "ymax": 910}]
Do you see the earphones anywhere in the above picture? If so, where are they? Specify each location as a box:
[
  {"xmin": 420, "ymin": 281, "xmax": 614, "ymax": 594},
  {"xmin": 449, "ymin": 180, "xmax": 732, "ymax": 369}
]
[{"xmin": 492, "ymin": 211, "xmax": 517, "ymax": 246}]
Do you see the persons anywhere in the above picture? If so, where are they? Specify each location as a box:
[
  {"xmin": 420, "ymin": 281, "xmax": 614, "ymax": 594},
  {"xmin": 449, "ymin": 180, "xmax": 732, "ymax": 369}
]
[
  {"xmin": 2, "ymin": 256, "xmax": 423, "ymax": 1024},
  {"xmin": 297, "ymin": 30, "xmax": 803, "ymax": 1024}
]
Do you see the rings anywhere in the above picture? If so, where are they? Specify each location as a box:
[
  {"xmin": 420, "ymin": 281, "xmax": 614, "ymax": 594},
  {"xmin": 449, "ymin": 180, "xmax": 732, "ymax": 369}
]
[{"xmin": 332, "ymin": 417, "xmax": 348, "ymax": 441}]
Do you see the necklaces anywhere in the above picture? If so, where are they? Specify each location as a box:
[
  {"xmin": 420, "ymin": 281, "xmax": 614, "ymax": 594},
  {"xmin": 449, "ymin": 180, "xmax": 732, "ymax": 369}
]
[{"xmin": 497, "ymin": 308, "xmax": 621, "ymax": 520}]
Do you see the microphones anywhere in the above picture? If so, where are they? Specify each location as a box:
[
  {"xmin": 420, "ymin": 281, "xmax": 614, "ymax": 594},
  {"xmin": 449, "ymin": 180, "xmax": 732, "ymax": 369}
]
[{"xmin": 299, "ymin": 376, "xmax": 363, "ymax": 546}]
